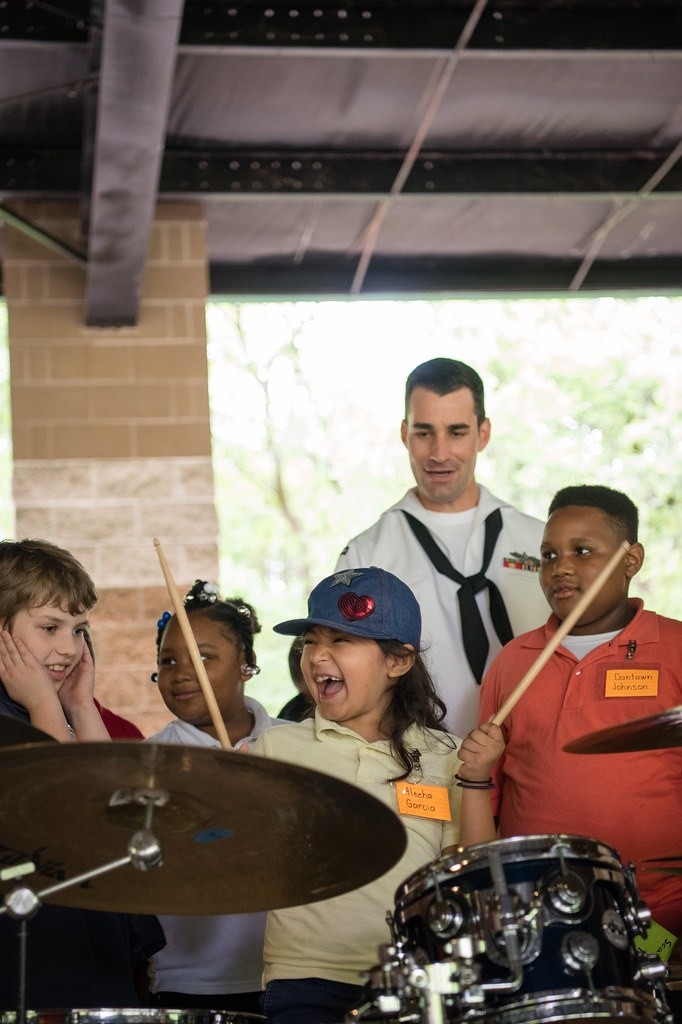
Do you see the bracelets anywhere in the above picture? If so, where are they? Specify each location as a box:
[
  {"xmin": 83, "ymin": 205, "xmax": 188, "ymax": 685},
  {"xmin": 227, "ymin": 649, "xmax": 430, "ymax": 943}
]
[
  {"xmin": 457, "ymin": 783, "xmax": 496, "ymax": 789},
  {"xmin": 455, "ymin": 774, "xmax": 492, "ymax": 784}
]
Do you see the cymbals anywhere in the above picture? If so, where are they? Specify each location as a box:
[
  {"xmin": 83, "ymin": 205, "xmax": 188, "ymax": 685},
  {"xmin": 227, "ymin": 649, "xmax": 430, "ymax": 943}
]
[
  {"xmin": 562, "ymin": 700, "xmax": 682, "ymax": 756},
  {"xmin": 0, "ymin": 737, "xmax": 411, "ymax": 918}
]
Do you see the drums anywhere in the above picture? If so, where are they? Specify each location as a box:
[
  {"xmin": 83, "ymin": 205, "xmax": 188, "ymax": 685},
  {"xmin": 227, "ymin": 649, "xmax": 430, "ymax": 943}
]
[
  {"xmin": 389, "ymin": 831, "xmax": 664, "ymax": 1023},
  {"xmin": 69, "ymin": 1001, "xmax": 282, "ymax": 1024}
]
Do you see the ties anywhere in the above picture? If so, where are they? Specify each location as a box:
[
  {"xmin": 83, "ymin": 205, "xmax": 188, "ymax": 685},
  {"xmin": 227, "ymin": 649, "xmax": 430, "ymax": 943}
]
[{"xmin": 401, "ymin": 508, "xmax": 515, "ymax": 685}]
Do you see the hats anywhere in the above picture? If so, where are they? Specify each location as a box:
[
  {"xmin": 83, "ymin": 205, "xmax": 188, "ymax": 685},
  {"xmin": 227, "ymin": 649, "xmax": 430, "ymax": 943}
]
[{"xmin": 273, "ymin": 567, "xmax": 422, "ymax": 652}]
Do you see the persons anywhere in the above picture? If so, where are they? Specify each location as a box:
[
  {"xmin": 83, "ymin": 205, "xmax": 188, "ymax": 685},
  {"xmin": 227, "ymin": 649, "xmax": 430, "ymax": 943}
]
[
  {"xmin": 0, "ymin": 541, "xmax": 297, "ymax": 1014},
  {"xmin": 233, "ymin": 568, "xmax": 504, "ymax": 1024},
  {"xmin": 476, "ymin": 485, "xmax": 682, "ymax": 930},
  {"xmin": 334, "ymin": 359, "xmax": 555, "ymax": 740}
]
